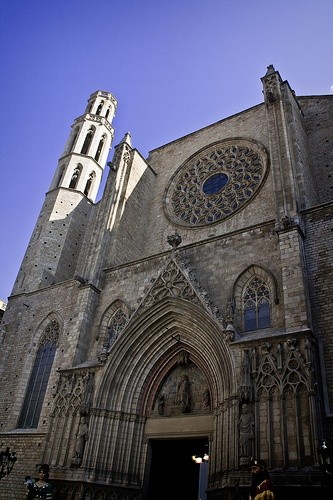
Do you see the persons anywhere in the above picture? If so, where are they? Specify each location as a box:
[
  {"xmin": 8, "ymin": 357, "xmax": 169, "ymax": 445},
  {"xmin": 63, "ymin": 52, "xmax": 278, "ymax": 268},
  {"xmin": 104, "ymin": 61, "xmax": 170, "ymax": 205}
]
[
  {"xmin": 31, "ymin": 464, "xmax": 56, "ymax": 500},
  {"xmin": 175, "ymin": 375, "xmax": 189, "ymax": 405},
  {"xmin": 24, "ymin": 476, "xmax": 34, "ymax": 500},
  {"xmin": 248, "ymin": 459, "xmax": 275, "ymax": 500},
  {"xmin": 72, "ymin": 416, "xmax": 88, "ymax": 458},
  {"xmin": 238, "ymin": 403, "xmax": 255, "ymax": 458}
]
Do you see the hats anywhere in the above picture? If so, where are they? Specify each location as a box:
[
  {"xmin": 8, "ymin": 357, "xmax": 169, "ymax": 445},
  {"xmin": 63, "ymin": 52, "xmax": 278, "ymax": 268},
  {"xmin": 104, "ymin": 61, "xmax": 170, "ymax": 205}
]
[{"xmin": 252, "ymin": 459, "xmax": 268, "ymax": 469}]
